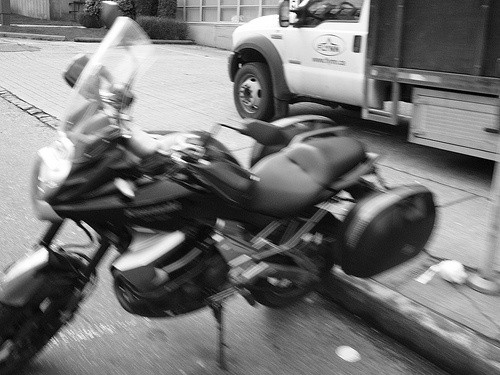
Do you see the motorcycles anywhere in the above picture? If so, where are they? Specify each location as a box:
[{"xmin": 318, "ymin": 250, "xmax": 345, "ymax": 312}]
[{"xmin": 0, "ymin": 16, "xmax": 435, "ymax": 375}]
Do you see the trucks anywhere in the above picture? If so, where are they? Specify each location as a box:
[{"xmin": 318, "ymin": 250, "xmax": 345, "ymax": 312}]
[{"xmin": 230, "ymin": 0, "xmax": 500, "ymax": 174}]
